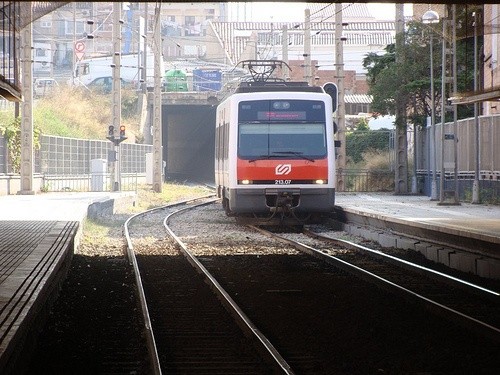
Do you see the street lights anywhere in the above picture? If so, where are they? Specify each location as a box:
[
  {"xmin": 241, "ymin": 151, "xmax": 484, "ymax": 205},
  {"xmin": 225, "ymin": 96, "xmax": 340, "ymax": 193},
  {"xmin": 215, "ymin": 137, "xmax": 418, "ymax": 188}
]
[{"xmin": 423, "ymin": 10, "xmax": 440, "ymax": 203}]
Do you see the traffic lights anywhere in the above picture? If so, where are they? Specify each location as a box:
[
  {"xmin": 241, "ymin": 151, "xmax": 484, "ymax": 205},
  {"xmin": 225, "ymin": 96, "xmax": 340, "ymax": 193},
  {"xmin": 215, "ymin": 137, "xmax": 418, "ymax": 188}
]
[
  {"xmin": 119, "ymin": 126, "xmax": 125, "ymax": 139},
  {"xmin": 108, "ymin": 125, "xmax": 115, "ymax": 139}
]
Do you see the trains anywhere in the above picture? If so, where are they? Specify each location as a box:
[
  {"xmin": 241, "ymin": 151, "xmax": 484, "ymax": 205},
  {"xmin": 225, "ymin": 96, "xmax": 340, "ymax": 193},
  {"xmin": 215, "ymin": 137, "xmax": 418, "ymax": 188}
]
[{"xmin": 215, "ymin": 77, "xmax": 338, "ymax": 226}]
